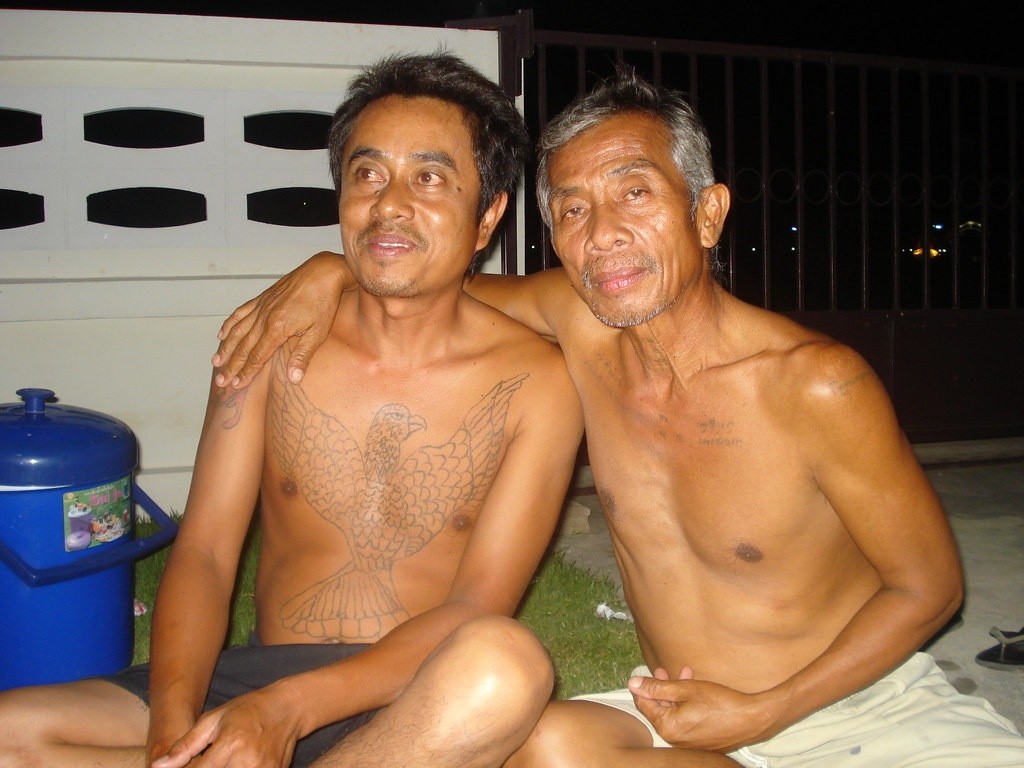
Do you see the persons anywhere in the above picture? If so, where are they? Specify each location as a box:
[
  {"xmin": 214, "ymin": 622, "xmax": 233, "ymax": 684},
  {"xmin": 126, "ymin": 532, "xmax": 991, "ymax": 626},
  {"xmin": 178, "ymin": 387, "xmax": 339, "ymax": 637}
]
[
  {"xmin": 0, "ymin": 52, "xmax": 583, "ymax": 768},
  {"xmin": 212, "ymin": 66, "xmax": 1024, "ymax": 768}
]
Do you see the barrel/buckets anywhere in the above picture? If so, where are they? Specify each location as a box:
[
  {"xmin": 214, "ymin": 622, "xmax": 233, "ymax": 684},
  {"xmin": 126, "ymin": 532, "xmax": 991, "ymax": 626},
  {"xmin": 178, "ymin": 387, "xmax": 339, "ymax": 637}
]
[{"xmin": 0, "ymin": 389, "xmax": 178, "ymax": 691}]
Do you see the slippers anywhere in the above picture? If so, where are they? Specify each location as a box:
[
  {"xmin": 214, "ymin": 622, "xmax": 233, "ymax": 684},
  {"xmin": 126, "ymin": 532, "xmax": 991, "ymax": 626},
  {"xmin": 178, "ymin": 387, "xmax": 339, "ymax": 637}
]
[{"xmin": 973, "ymin": 627, "xmax": 1024, "ymax": 672}]
